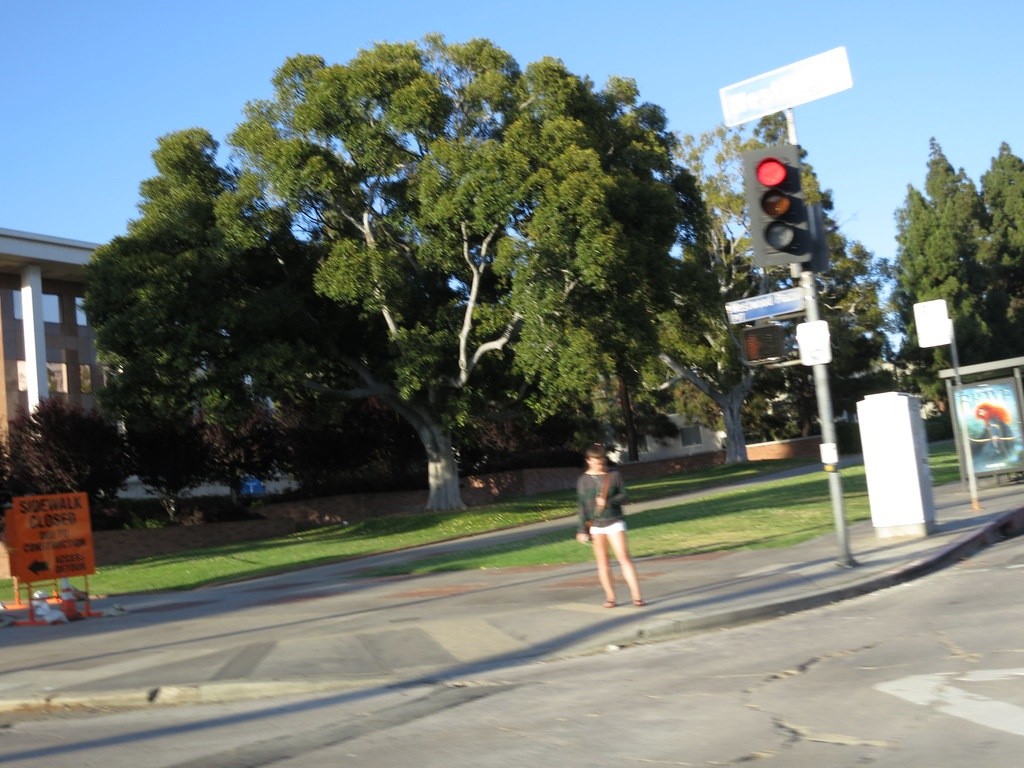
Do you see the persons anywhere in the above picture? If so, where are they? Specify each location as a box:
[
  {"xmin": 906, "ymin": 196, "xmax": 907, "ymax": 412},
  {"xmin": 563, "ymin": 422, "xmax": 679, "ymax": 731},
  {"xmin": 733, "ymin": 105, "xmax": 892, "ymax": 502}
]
[{"xmin": 576, "ymin": 442, "xmax": 644, "ymax": 609}]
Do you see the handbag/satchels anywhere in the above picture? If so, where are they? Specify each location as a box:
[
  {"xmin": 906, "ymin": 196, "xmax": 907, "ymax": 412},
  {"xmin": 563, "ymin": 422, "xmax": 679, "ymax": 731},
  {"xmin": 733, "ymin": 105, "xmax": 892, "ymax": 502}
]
[{"xmin": 584, "ymin": 519, "xmax": 592, "ymax": 531}]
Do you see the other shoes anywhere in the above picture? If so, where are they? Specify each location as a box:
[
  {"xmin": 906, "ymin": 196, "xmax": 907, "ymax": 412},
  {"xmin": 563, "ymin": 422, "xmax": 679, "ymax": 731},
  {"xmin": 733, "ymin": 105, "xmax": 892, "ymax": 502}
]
[
  {"xmin": 604, "ymin": 601, "xmax": 617, "ymax": 607},
  {"xmin": 632, "ymin": 598, "xmax": 643, "ymax": 606}
]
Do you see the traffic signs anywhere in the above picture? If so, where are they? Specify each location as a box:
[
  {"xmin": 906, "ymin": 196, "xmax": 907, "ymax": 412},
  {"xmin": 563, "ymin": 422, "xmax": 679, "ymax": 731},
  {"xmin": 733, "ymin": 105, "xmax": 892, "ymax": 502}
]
[{"xmin": 12, "ymin": 493, "xmax": 95, "ymax": 584}]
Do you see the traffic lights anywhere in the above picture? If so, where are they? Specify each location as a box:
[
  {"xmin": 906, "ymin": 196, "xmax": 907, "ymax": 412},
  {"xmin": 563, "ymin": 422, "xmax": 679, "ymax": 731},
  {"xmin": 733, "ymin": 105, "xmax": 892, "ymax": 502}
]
[
  {"xmin": 739, "ymin": 324, "xmax": 786, "ymax": 366},
  {"xmin": 742, "ymin": 145, "xmax": 812, "ymax": 265}
]
